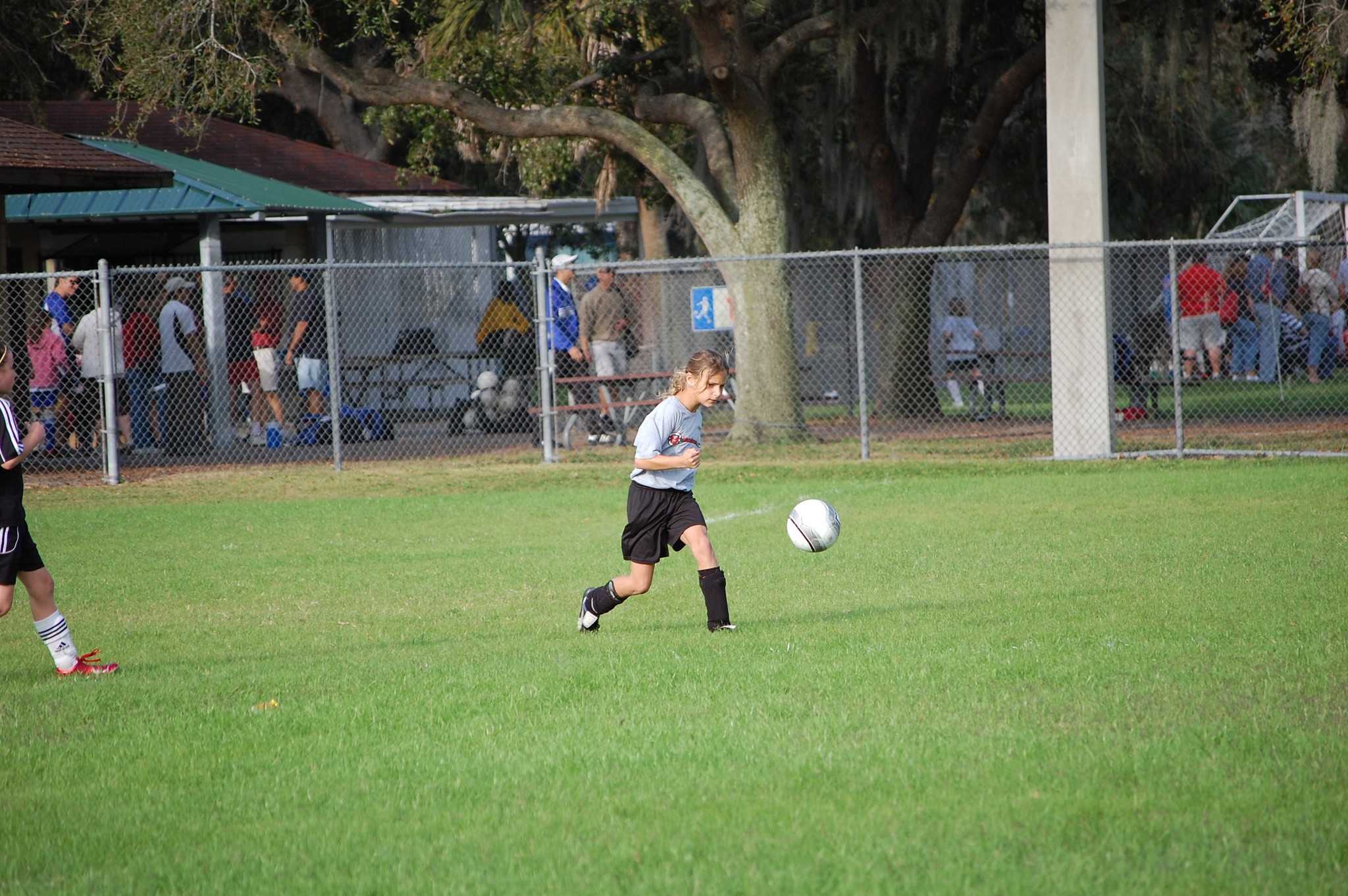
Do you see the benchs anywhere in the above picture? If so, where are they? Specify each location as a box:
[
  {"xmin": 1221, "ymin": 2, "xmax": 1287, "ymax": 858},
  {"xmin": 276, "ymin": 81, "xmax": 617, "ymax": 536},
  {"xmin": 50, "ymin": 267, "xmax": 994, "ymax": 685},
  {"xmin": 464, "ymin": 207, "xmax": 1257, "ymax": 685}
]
[
  {"xmin": 950, "ymin": 372, "xmax": 1201, "ymax": 418},
  {"xmin": 346, "ymin": 376, "xmax": 469, "ymax": 413},
  {"xmin": 530, "ymin": 397, "xmax": 736, "ymax": 449}
]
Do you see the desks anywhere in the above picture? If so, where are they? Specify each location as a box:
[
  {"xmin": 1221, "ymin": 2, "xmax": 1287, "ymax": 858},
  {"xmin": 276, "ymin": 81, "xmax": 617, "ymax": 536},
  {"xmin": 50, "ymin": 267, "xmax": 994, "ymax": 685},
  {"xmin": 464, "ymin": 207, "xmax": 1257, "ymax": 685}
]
[
  {"xmin": 349, "ymin": 351, "xmax": 495, "ymax": 413},
  {"xmin": 552, "ymin": 366, "xmax": 809, "ymax": 444},
  {"xmin": 935, "ymin": 345, "xmax": 1193, "ymax": 413}
]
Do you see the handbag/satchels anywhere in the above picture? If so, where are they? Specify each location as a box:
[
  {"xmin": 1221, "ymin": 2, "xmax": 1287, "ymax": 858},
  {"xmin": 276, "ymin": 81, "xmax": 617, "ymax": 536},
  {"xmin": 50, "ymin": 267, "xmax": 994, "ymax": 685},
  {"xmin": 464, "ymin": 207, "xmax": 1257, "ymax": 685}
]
[
  {"xmin": 317, "ymin": 402, "xmax": 394, "ymax": 442},
  {"xmin": 624, "ymin": 328, "xmax": 639, "ymax": 359}
]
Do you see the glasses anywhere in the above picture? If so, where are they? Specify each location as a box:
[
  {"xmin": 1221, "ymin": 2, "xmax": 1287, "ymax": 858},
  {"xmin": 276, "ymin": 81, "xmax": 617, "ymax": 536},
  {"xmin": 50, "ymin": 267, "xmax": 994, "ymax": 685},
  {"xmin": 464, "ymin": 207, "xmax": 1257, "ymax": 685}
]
[{"xmin": 61, "ymin": 278, "xmax": 77, "ymax": 285}]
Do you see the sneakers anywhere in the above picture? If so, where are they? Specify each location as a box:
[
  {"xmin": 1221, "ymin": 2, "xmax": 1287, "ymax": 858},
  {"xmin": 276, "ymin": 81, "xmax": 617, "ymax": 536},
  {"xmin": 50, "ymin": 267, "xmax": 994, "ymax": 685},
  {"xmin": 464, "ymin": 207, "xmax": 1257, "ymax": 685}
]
[
  {"xmin": 588, "ymin": 432, "xmax": 613, "ymax": 446},
  {"xmin": 578, "ymin": 587, "xmax": 600, "ymax": 634},
  {"xmin": 54, "ymin": 648, "xmax": 119, "ymax": 677},
  {"xmin": 531, "ymin": 438, "xmax": 561, "ymax": 449}
]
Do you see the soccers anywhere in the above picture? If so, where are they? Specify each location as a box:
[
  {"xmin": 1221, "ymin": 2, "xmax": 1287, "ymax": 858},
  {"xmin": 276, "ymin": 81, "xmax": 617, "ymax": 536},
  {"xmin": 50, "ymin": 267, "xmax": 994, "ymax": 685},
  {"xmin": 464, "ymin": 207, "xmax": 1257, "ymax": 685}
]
[
  {"xmin": 786, "ymin": 499, "xmax": 840, "ymax": 552},
  {"xmin": 462, "ymin": 370, "xmax": 523, "ymax": 429}
]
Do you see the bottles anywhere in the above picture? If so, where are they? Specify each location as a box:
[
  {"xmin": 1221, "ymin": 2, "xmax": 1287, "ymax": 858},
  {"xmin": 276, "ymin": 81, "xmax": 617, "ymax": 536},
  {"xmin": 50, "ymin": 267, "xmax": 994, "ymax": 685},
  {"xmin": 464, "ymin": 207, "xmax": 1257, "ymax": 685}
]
[
  {"xmin": 1168, "ymin": 364, "xmax": 1174, "ymax": 380},
  {"xmin": 1150, "ymin": 361, "xmax": 1160, "ymax": 380}
]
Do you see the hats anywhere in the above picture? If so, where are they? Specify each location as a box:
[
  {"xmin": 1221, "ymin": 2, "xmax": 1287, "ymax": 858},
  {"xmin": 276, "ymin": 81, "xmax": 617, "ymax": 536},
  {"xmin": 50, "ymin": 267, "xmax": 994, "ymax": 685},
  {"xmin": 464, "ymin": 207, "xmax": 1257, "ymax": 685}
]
[
  {"xmin": 550, "ymin": 253, "xmax": 578, "ymax": 267},
  {"xmin": 163, "ymin": 277, "xmax": 195, "ymax": 293}
]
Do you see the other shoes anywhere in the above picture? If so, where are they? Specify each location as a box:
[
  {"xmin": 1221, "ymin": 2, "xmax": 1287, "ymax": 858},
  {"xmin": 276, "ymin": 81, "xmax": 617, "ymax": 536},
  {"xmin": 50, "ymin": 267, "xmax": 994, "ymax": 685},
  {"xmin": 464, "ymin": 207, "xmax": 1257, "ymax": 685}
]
[
  {"xmin": 1232, "ymin": 375, "xmax": 1238, "ymax": 383},
  {"xmin": 1211, "ymin": 372, "xmax": 1221, "ymax": 380},
  {"xmin": 1247, "ymin": 374, "xmax": 1259, "ymax": 381},
  {"xmin": 248, "ymin": 433, "xmax": 265, "ymax": 445}
]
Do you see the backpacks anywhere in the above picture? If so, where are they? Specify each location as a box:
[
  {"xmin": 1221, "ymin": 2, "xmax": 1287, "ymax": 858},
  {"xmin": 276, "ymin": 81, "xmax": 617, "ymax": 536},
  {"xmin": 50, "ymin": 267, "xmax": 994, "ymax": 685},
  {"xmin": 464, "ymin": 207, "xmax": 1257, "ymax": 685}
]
[
  {"xmin": 1219, "ymin": 290, "xmax": 1238, "ymax": 322},
  {"xmin": 1291, "ymin": 272, "xmax": 1328, "ymax": 315}
]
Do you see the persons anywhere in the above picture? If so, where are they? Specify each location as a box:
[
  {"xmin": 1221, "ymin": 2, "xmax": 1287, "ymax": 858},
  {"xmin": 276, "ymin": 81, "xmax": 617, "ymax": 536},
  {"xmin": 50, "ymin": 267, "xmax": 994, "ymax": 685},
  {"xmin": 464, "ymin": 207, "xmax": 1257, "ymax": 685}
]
[
  {"xmin": 943, "ymin": 296, "xmax": 984, "ymax": 408},
  {"xmin": 578, "ymin": 266, "xmax": 631, "ymax": 416},
  {"xmin": 1164, "ymin": 244, "xmax": 1348, "ymax": 385},
  {"xmin": 532, "ymin": 253, "xmax": 616, "ymax": 452},
  {"xmin": 25, "ymin": 271, "xmax": 328, "ymax": 455},
  {"xmin": 578, "ymin": 350, "xmax": 736, "ymax": 633},
  {"xmin": 0, "ymin": 343, "xmax": 121, "ymax": 680}
]
